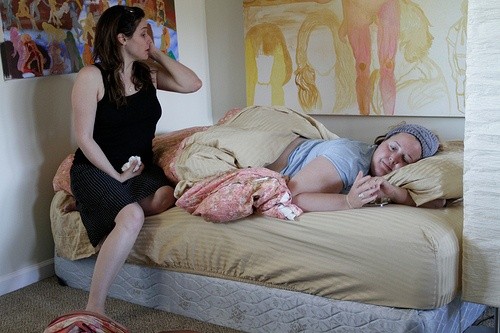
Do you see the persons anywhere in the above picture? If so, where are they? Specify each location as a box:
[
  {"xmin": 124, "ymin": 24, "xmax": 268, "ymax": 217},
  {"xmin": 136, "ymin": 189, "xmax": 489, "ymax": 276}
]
[
  {"xmin": 219, "ymin": 105, "xmax": 446, "ymax": 212},
  {"xmin": 70, "ymin": 5, "xmax": 203, "ymax": 314}
]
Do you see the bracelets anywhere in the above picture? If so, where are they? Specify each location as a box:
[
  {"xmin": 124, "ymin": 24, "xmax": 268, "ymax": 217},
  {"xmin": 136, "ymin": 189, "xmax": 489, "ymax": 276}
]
[{"xmin": 346, "ymin": 194, "xmax": 352, "ymax": 210}]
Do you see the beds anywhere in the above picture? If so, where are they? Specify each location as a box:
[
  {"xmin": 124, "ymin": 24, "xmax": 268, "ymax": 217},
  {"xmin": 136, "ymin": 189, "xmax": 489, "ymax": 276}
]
[{"xmin": 48, "ymin": 190, "xmax": 486, "ymax": 333}]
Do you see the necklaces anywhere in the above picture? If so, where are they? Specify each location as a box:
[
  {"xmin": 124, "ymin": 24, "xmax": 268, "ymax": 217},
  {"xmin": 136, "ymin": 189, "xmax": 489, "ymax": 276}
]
[{"xmin": 122, "ymin": 77, "xmax": 132, "ymax": 92}]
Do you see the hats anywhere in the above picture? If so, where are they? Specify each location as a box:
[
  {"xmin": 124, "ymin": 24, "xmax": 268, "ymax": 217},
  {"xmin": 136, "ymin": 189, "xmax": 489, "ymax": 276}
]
[{"xmin": 384, "ymin": 124, "xmax": 439, "ymax": 158}]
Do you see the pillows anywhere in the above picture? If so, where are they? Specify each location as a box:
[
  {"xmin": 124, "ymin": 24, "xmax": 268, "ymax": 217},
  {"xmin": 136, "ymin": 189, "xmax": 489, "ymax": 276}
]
[{"xmin": 382, "ymin": 140, "xmax": 464, "ymax": 207}]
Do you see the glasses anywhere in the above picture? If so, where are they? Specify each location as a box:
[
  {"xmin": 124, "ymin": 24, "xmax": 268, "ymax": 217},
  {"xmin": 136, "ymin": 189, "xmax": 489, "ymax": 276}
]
[{"xmin": 117, "ymin": 7, "xmax": 135, "ymax": 33}]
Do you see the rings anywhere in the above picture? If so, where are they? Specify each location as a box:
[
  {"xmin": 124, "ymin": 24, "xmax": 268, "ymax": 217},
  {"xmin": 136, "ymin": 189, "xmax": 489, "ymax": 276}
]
[{"xmin": 359, "ymin": 193, "xmax": 363, "ymax": 198}]
